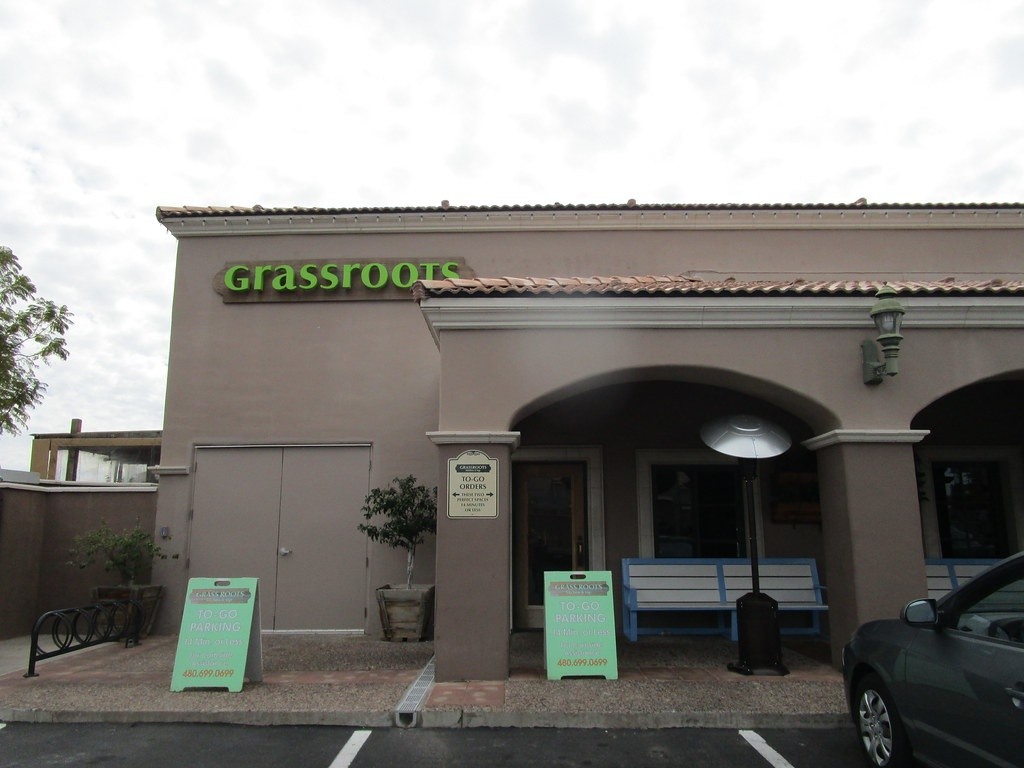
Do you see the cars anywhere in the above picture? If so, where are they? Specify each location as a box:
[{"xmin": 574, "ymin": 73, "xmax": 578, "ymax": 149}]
[{"xmin": 842, "ymin": 551, "xmax": 1024, "ymax": 768}]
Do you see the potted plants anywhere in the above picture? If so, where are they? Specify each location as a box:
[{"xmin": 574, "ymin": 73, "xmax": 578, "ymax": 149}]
[
  {"xmin": 355, "ymin": 473, "xmax": 438, "ymax": 643},
  {"xmin": 66, "ymin": 514, "xmax": 180, "ymax": 640}
]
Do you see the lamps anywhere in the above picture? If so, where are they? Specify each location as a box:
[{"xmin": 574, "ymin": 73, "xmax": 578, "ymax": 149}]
[{"xmin": 868, "ymin": 280, "xmax": 906, "ymax": 377}]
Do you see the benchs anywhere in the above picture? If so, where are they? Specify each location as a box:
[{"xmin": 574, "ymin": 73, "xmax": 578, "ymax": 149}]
[
  {"xmin": 620, "ymin": 557, "xmax": 827, "ymax": 642},
  {"xmin": 924, "ymin": 558, "xmax": 1024, "ymax": 612}
]
